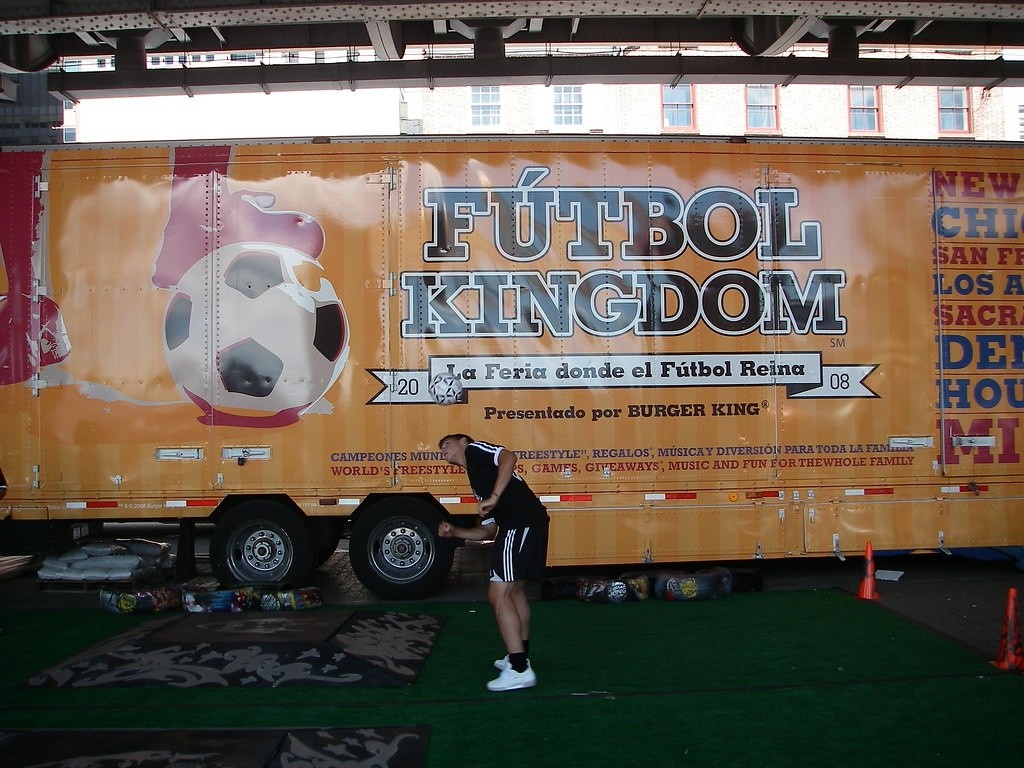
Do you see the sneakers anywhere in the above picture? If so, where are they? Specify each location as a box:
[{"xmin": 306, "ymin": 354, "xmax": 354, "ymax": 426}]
[
  {"xmin": 493, "ymin": 656, "xmax": 510, "ymax": 670},
  {"xmin": 486, "ymin": 660, "xmax": 536, "ymax": 691}
]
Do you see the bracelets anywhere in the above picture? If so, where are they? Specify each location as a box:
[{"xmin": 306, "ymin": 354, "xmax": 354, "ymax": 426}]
[{"xmin": 490, "ymin": 489, "xmax": 500, "ymax": 499}]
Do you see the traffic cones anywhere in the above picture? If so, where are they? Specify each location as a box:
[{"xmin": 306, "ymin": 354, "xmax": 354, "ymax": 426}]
[
  {"xmin": 989, "ymin": 589, "xmax": 1024, "ymax": 672},
  {"xmin": 856, "ymin": 541, "xmax": 882, "ymax": 598}
]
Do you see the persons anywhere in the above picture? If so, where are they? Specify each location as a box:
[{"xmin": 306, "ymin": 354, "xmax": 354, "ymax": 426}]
[{"xmin": 437, "ymin": 432, "xmax": 551, "ymax": 692}]
